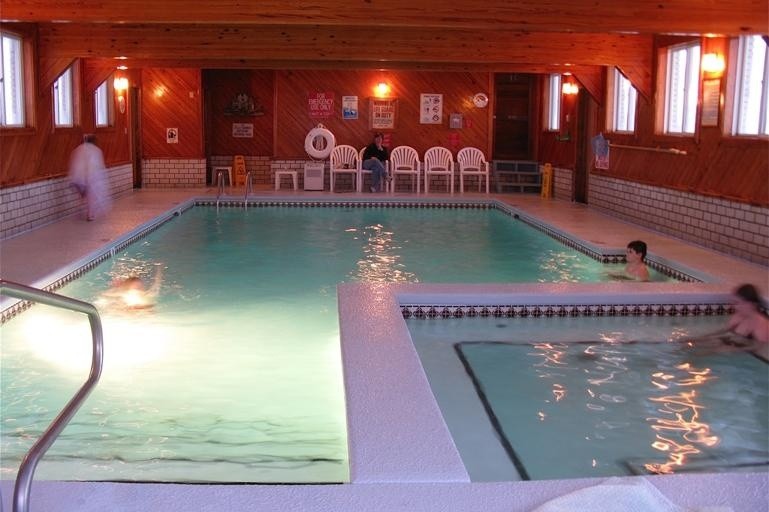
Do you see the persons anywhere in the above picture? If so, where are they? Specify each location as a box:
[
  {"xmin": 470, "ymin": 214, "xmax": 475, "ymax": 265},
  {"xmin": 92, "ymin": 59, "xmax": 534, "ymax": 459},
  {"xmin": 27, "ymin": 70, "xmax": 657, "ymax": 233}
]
[
  {"xmin": 362, "ymin": 132, "xmax": 393, "ymax": 193},
  {"xmin": 705, "ymin": 283, "xmax": 769, "ymax": 355},
  {"xmin": 607, "ymin": 240, "xmax": 650, "ymax": 283},
  {"xmin": 67, "ymin": 133, "xmax": 108, "ymax": 222},
  {"xmin": 101, "ymin": 269, "xmax": 161, "ymax": 311}
]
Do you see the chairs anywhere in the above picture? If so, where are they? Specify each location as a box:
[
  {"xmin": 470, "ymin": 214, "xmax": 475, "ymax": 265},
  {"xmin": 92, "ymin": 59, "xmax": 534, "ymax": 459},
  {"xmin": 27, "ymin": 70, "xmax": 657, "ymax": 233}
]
[{"xmin": 328, "ymin": 145, "xmax": 491, "ymax": 194}]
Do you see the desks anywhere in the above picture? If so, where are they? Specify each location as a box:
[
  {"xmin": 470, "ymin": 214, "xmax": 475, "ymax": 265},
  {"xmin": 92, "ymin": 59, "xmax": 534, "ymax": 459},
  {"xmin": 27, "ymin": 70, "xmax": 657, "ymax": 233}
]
[
  {"xmin": 274, "ymin": 169, "xmax": 298, "ymax": 191},
  {"xmin": 211, "ymin": 166, "xmax": 233, "ymax": 187}
]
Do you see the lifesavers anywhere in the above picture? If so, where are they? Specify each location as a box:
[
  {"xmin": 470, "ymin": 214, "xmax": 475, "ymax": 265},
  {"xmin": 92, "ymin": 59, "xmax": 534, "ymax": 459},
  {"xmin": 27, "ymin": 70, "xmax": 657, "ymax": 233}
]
[{"xmin": 305, "ymin": 128, "xmax": 335, "ymax": 158}]
